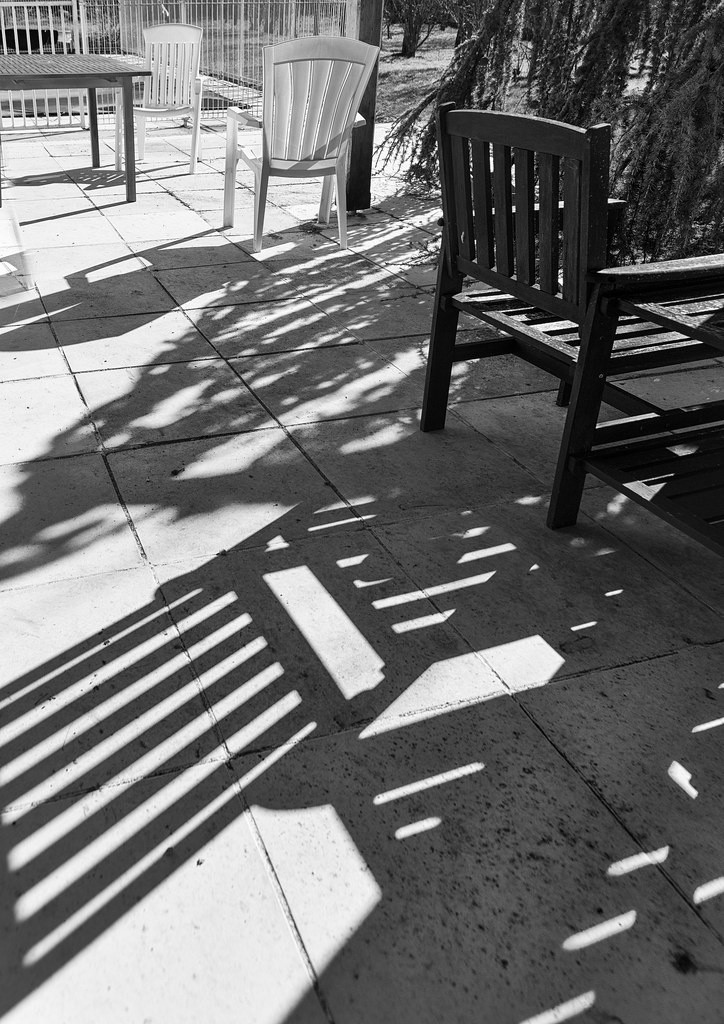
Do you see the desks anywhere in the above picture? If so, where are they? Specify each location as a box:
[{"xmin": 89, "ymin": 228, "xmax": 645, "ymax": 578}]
[{"xmin": 0, "ymin": 54, "xmax": 152, "ymax": 207}]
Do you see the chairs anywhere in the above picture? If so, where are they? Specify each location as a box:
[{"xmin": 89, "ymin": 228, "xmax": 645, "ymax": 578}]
[
  {"xmin": 224, "ymin": 35, "xmax": 381, "ymax": 252},
  {"xmin": 115, "ymin": 24, "xmax": 205, "ymax": 174},
  {"xmin": 420, "ymin": 102, "xmax": 724, "ymax": 531}
]
